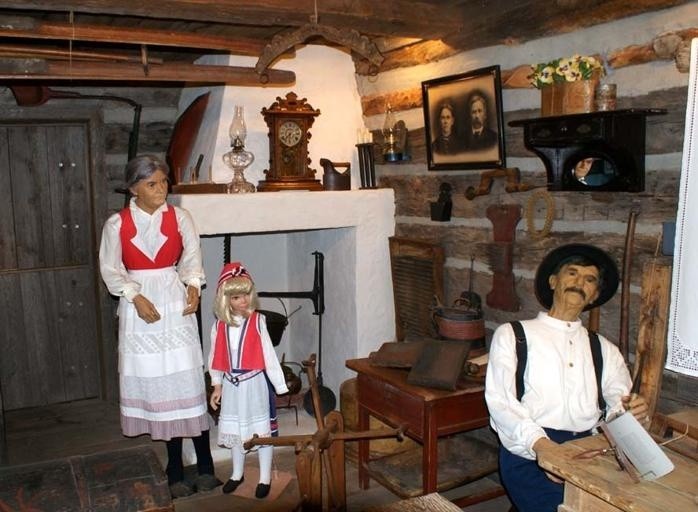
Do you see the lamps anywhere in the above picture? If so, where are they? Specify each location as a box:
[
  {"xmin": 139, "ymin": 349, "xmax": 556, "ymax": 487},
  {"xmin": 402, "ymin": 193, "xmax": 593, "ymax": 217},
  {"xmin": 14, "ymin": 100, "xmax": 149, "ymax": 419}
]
[
  {"xmin": 220, "ymin": 103, "xmax": 256, "ymax": 196},
  {"xmin": 381, "ymin": 96, "xmax": 412, "ymax": 162}
]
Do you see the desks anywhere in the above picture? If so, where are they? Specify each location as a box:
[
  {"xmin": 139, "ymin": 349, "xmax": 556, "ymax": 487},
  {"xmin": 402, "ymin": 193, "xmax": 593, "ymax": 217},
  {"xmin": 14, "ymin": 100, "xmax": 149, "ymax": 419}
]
[
  {"xmin": 536, "ymin": 429, "xmax": 698, "ymax": 512},
  {"xmin": 343, "ymin": 355, "xmax": 507, "ymax": 508}
]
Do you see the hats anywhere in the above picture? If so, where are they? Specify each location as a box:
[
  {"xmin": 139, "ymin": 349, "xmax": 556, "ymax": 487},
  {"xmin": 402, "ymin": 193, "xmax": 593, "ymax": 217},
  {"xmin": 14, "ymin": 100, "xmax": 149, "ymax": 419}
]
[
  {"xmin": 534, "ymin": 243, "xmax": 619, "ymax": 312},
  {"xmin": 216, "ymin": 261, "xmax": 254, "ymax": 294}
]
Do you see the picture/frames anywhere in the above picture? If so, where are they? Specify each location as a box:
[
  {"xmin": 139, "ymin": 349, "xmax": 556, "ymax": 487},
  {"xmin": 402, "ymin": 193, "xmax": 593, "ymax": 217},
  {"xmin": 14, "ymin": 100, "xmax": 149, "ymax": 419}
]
[{"xmin": 419, "ymin": 63, "xmax": 510, "ymax": 171}]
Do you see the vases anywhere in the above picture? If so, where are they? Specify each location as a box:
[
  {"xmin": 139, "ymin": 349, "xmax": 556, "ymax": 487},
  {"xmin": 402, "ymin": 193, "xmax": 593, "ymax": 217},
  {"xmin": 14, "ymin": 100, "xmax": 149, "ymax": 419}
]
[{"xmin": 540, "ymin": 77, "xmax": 595, "ymax": 119}]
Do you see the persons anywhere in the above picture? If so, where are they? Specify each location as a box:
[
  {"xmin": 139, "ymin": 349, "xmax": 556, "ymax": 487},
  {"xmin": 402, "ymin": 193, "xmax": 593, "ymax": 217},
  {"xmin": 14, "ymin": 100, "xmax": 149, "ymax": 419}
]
[
  {"xmin": 97, "ymin": 155, "xmax": 224, "ymax": 491},
  {"xmin": 431, "ymin": 103, "xmax": 464, "ymax": 154},
  {"xmin": 572, "ymin": 157, "xmax": 593, "ymax": 186},
  {"xmin": 466, "ymin": 94, "xmax": 497, "ymax": 149},
  {"xmin": 483, "ymin": 244, "xmax": 652, "ymax": 512},
  {"xmin": 206, "ymin": 262, "xmax": 290, "ymax": 500}
]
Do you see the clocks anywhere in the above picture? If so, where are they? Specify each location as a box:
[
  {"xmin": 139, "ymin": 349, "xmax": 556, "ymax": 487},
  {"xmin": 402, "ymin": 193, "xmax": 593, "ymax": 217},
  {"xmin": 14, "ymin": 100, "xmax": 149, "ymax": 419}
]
[{"xmin": 256, "ymin": 89, "xmax": 324, "ymax": 191}]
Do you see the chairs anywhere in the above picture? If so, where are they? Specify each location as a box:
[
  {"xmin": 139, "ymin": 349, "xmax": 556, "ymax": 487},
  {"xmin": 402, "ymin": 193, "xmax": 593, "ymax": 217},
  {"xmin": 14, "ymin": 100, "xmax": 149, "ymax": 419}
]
[{"xmin": 293, "ymin": 408, "xmax": 466, "ymax": 512}]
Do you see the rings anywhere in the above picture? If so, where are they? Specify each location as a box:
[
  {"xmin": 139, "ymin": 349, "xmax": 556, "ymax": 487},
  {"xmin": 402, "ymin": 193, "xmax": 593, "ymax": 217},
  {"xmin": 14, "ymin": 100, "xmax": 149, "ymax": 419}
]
[{"xmin": 644, "ymin": 416, "xmax": 649, "ymax": 423}]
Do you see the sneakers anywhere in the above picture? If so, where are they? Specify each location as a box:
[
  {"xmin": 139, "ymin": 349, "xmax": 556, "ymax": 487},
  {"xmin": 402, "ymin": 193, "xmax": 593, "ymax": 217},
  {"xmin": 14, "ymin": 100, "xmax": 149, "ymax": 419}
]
[{"xmin": 165, "ymin": 467, "xmax": 272, "ymax": 498}]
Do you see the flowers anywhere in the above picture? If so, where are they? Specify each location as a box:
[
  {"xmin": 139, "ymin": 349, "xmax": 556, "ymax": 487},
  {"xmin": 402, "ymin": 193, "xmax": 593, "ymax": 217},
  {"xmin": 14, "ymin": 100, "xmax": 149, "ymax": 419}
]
[{"xmin": 524, "ymin": 53, "xmax": 606, "ymax": 88}]
